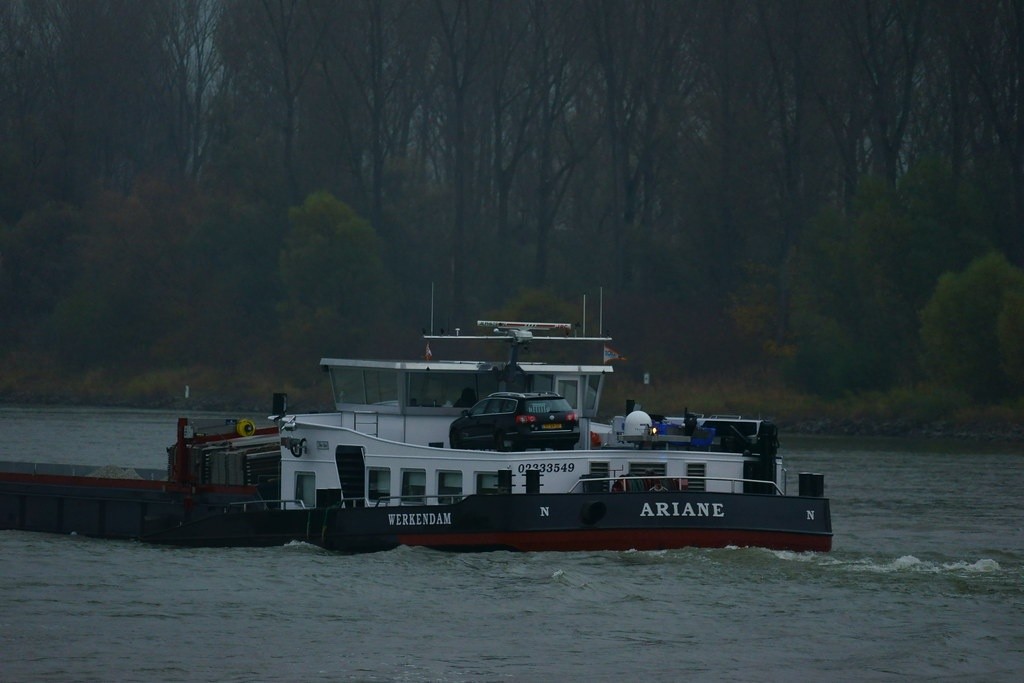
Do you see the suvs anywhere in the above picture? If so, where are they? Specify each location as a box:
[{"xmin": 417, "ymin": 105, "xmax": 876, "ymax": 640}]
[{"xmin": 447, "ymin": 391, "xmax": 582, "ymax": 452}]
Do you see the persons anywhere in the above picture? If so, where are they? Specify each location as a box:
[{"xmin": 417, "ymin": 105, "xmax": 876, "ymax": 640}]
[{"xmin": 410, "ymin": 399, "xmax": 416, "ymax": 407}]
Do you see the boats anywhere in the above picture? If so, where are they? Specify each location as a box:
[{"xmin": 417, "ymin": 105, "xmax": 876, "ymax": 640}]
[{"xmin": 267, "ymin": 280, "xmax": 835, "ymax": 553}]
